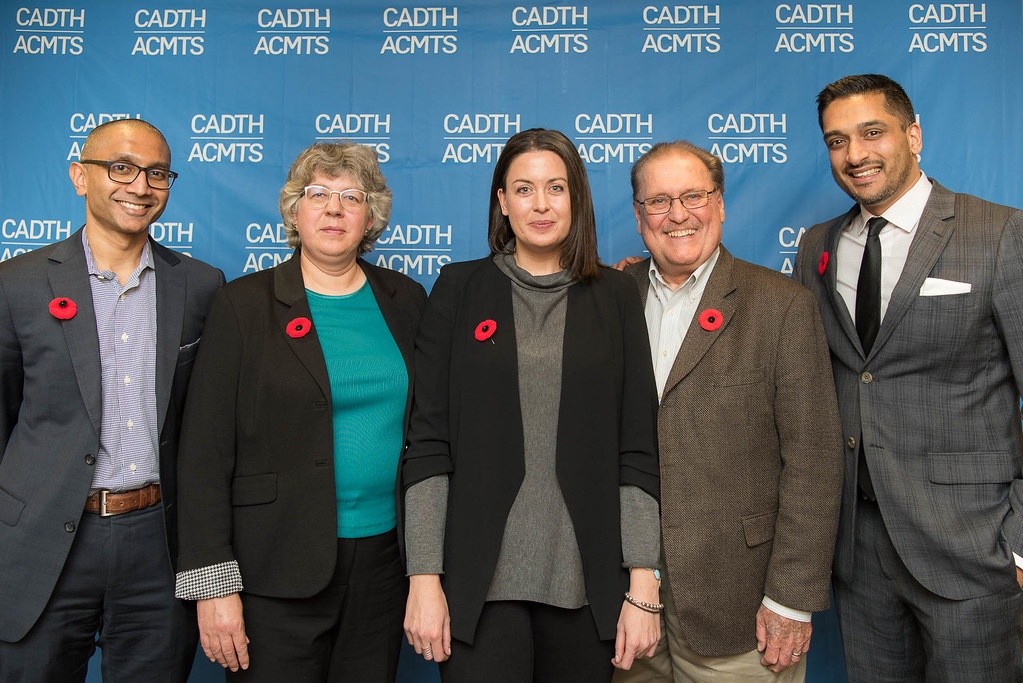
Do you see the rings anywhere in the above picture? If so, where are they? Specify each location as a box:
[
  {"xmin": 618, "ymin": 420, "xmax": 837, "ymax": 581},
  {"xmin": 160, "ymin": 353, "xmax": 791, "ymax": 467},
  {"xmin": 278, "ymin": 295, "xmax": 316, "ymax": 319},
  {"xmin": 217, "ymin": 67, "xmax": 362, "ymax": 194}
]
[{"xmin": 792, "ymin": 653, "xmax": 801, "ymax": 656}]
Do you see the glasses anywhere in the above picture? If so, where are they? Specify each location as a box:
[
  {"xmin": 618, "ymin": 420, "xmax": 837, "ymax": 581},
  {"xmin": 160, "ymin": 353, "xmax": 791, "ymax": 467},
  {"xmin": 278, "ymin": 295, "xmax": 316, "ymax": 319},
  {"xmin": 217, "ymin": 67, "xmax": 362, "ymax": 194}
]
[
  {"xmin": 300, "ymin": 185, "xmax": 368, "ymax": 210},
  {"xmin": 637, "ymin": 188, "xmax": 716, "ymax": 215},
  {"xmin": 80, "ymin": 160, "xmax": 178, "ymax": 190}
]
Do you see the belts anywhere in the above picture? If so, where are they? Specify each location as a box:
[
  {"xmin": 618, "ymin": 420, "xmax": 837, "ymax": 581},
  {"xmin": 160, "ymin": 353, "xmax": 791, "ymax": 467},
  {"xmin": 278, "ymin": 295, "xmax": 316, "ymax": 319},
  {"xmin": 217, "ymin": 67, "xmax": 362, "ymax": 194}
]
[
  {"xmin": 84, "ymin": 485, "xmax": 161, "ymax": 516},
  {"xmin": 857, "ymin": 485, "xmax": 875, "ymax": 506}
]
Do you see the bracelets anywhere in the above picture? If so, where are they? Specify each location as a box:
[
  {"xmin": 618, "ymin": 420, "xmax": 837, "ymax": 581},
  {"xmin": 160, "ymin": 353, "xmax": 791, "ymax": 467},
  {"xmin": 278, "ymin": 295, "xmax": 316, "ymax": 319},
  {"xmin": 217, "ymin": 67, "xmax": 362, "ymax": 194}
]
[
  {"xmin": 625, "ymin": 592, "xmax": 664, "ymax": 613},
  {"xmin": 629, "ymin": 567, "xmax": 662, "ymax": 586}
]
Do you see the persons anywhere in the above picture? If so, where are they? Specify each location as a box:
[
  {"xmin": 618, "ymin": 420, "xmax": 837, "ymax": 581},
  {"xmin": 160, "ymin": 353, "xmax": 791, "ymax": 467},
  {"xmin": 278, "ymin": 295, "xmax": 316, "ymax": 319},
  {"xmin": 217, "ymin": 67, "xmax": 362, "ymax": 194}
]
[
  {"xmin": 174, "ymin": 142, "xmax": 429, "ymax": 683},
  {"xmin": 619, "ymin": 142, "xmax": 846, "ymax": 682},
  {"xmin": 1, "ymin": 118, "xmax": 229, "ymax": 683},
  {"xmin": 610, "ymin": 74, "xmax": 1023, "ymax": 683},
  {"xmin": 396, "ymin": 125, "xmax": 665, "ymax": 683}
]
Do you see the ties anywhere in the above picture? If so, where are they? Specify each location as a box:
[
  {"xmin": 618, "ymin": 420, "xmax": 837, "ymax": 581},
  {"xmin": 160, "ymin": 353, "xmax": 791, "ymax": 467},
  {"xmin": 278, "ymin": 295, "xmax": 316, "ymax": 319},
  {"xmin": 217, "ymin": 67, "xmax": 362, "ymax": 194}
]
[{"xmin": 855, "ymin": 217, "xmax": 889, "ymax": 501}]
